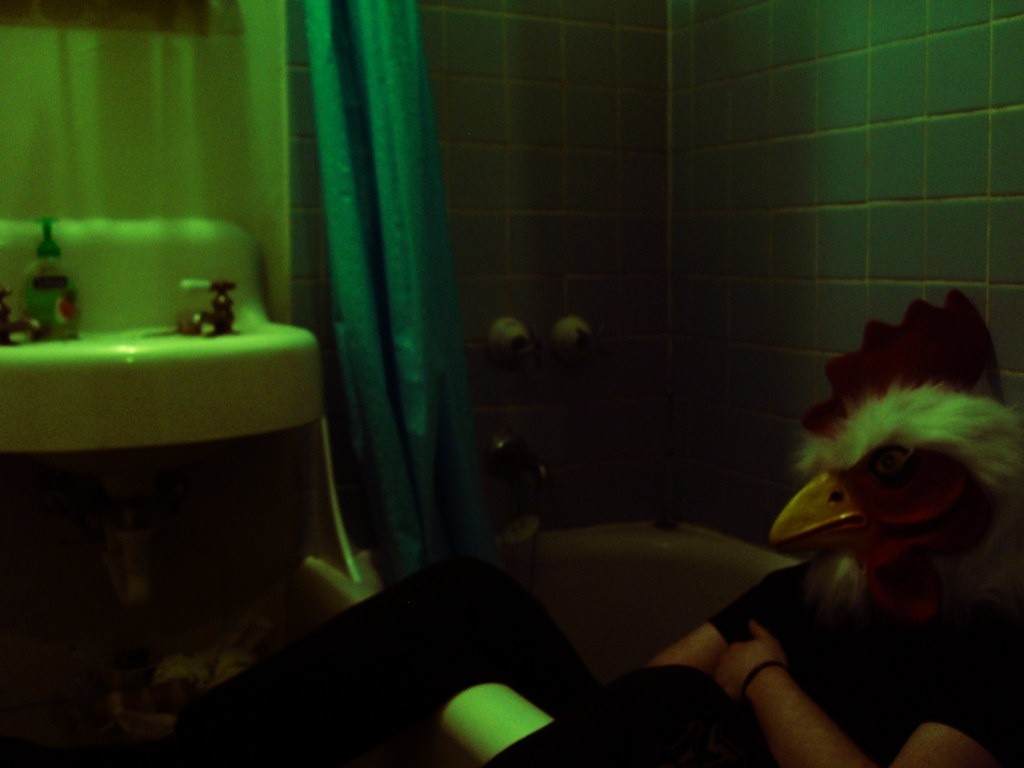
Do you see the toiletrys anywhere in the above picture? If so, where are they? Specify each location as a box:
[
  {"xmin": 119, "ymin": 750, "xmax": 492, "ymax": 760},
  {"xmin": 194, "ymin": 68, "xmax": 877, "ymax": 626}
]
[{"xmin": 20, "ymin": 215, "xmax": 80, "ymax": 341}]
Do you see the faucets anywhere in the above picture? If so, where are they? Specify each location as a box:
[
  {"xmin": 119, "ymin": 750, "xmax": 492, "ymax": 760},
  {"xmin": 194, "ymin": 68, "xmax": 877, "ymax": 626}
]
[
  {"xmin": 489, "ymin": 433, "xmax": 548, "ymax": 486},
  {"xmin": 177, "ymin": 281, "xmax": 237, "ymax": 336}
]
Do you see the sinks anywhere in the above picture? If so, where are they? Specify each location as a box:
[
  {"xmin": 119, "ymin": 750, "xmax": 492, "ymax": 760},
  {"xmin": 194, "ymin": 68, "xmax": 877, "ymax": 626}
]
[{"xmin": 0, "ymin": 215, "xmax": 321, "ymax": 478}]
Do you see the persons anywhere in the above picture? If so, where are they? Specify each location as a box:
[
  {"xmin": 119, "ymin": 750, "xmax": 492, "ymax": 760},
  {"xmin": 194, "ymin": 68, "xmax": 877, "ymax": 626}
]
[{"xmin": 154, "ymin": 278, "xmax": 1024, "ymax": 767}]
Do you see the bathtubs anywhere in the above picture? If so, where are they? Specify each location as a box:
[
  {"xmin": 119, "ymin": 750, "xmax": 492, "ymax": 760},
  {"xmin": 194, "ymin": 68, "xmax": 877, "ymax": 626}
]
[{"xmin": 297, "ymin": 517, "xmax": 804, "ymax": 768}]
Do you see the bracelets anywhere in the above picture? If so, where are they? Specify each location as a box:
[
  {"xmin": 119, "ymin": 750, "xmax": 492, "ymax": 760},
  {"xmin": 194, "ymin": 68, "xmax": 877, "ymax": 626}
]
[{"xmin": 740, "ymin": 660, "xmax": 788, "ymax": 699}]
